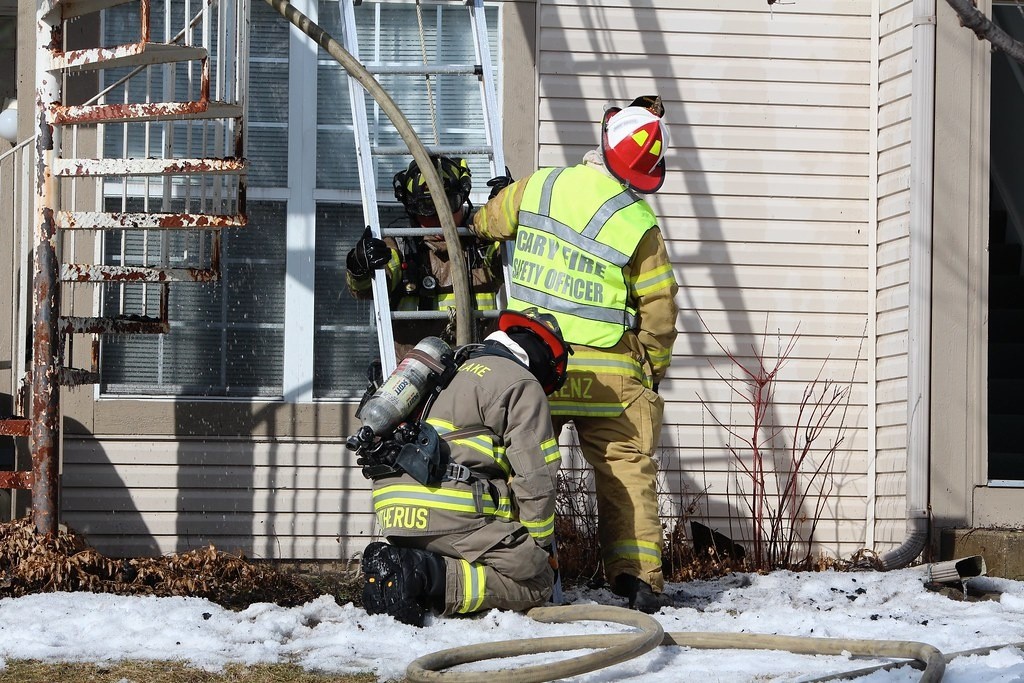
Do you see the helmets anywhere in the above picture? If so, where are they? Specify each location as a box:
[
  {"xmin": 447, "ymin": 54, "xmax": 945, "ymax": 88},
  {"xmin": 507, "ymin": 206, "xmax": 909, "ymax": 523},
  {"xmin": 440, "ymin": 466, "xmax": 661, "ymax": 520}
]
[
  {"xmin": 498, "ymin": 307, "xmax": 574, "ymax": 398},
  {"xmin": 601, "ymin": 106, "xmax": 670, "ymax": 194},
  {"xmin": 392, "ymin": 153, "xmax": 472, "ymax": 217}
]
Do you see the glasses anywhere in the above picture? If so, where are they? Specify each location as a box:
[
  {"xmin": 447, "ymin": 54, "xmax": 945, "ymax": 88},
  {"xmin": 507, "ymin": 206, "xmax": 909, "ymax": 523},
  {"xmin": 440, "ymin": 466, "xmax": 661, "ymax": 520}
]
[{"xmin": 410, "ymin": 193, "xmax": 463, "ymax": 216}]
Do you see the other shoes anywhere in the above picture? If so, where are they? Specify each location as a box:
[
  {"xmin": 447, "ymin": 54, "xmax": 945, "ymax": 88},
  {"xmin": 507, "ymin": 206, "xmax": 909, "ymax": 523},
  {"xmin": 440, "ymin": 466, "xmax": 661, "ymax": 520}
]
[{"xmin": 627, "ymin": 578, "xmax": 659, "ymax": 613}]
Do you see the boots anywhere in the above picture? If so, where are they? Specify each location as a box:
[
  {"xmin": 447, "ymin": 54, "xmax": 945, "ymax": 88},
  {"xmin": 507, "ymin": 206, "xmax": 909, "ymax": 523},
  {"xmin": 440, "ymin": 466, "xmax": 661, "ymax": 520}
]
[
  {"xmin": 360, "ymin": 542, "xmax": 399, "ymax": 616},
  {"xmin": 375, "ymin": 545, "xmax": 447, "ymax": 628}
]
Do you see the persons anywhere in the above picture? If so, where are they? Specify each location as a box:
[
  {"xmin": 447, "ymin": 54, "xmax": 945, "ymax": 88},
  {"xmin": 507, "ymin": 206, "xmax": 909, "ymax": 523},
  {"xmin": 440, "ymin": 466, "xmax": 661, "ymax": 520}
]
[
  {"xmin": 360, "ymin": 307, "xmax": 575, "ymax": 630},
  {"xmin": 474, "ymin": 94, "xmax": 682, "ymax": 609},
  {"xmin": 345, "ymin": 155, "xmax": 515, "ymax": 363}
]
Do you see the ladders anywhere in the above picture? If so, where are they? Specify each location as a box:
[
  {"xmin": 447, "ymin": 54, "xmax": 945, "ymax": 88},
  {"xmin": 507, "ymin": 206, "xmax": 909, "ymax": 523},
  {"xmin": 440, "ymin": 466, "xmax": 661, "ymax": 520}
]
[{"xmin": 337, "ymin": 1, "xmax": 513, "ymax": 388}]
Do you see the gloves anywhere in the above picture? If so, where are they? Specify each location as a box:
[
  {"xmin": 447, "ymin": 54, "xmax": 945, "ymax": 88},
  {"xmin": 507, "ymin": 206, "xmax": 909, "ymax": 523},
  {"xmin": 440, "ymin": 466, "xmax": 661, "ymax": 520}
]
[
  {"xmin": 346, "ymin": 226, "xmax": 392, "ymax": 281},
  {"xmin": 486, "ymin": 166, "xmax": 515, "ymax": 201},
  {"xmin": 652, "ymin": 382, "xmax": 659, "ymax": 394},
  {"xmin": 541, "ymin": 542, "xmax": 559, "ymax": 586}
]
